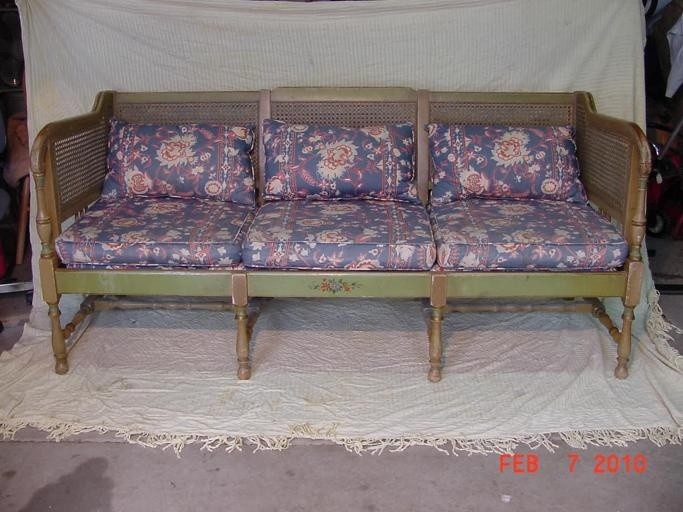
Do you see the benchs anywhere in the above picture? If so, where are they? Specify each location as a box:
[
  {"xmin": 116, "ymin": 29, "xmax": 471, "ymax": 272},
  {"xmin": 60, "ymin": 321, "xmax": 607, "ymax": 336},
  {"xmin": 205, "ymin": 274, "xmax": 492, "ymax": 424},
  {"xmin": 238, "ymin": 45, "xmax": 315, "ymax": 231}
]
[{"xmin": 30, "ymin": 87, "xmax": 653, "ymax": 383}]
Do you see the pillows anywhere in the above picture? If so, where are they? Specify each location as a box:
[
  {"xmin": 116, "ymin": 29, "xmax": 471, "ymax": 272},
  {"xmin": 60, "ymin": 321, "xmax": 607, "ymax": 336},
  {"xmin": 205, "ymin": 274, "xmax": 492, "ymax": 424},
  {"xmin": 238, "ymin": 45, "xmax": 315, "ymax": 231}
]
[
  {"xmin": 101, "ymin": 115, "xmax": 258, "ymax": 208},
  {"xmin": 262, "ymin": 118, "xmax": 423, "ymax": 205},
  {"xmin": 423, "ymin": 122, "xmax": 588, "ymax": 204}
]
